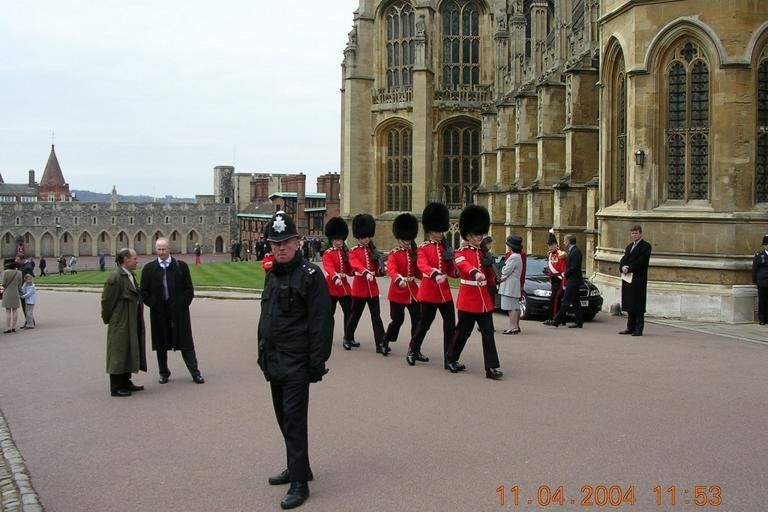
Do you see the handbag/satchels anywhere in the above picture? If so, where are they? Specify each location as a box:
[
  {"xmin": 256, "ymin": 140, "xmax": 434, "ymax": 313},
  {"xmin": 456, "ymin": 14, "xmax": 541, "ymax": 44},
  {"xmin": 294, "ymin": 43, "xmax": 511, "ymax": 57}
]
[
  {"xmin": 0, "ymin": 293, "xmax": 3, "ymax": 299},
  {"xmin": 519, "ymin": 297, "xmax": 526, "ymax": 317}
]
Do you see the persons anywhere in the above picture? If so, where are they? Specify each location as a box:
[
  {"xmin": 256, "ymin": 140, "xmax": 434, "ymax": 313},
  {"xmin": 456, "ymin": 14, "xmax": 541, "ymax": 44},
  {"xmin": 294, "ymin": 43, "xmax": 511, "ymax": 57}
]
[
  {"xmin": 499, "ymin": 235, "xmax": 524, "ymax": 334},
  {"xmin": 377, "ymin": 238, "xmax": 429, "ymax": 362},
  {"xmin": 542, "ymin": 242, "xmax": 567, "ymax": 325},
  {"xmin": 617, "ymin": 224, "xmax": 652, "ymax": 337},
  {"xmin": 1, "ymin": 257, "xmax": 22, "ymax": 333},
  {"xmin": 341, "ymin": 234, "xmax": 392, "ymax": 353},
  {"xmin": 752, "ymin": 243, "xmax": 768, "ymax": 325},
  {"xmin": 476, "ymin": 234, "xmax": 497, "ymax": 332},
  {"xmin": 100, "ymin": 247, "xmax": 148, "ymax": 397},
  {"xmin": 20, "ymin": 273, "xmax": 35, "ymax": 329},
  {"xmin": 227, "ymin": 236, "xmax": 324, "ymax": 262},
  {"xmin": 405, "ymin": 229, "xmax": 467, "ymax": 370},
  {"xmin": 28, "ymin": 251, "xmax": 107, "ymax": 278},
  {"xmin": 551, "ymin": 234, "xmax": 584, "ymax": 328},
  {"xmin": 444, "ymin": 229, "xmax": 509, "ymax": 380},
  {"xmin": 139, "ymin": 236, "xmax": 205, "ymax": 384},
  {"xmin": 14, "ymin": 253, "xmax": 36, "ymax": 329},
  {"xmin": 503, "ymin": 235, "xmax": 526, "ymax": 332},
  {"xmin": 193, "ymin": 242, "xmax": 202, "ymax": 265},
  {"xmin": 256, "ymin": 213, "xmax": 335, "ymax": 509},
  {"xmin": 322, "ymin": 236, "xmax": 361, "ymax": 347}
]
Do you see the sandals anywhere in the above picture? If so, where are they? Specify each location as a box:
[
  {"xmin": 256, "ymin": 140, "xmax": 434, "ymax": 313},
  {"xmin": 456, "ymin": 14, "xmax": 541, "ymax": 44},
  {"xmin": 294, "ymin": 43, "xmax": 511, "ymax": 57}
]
[{"xmin": 502, "ymin": 326, "xmax": 520, "ymax": 335}]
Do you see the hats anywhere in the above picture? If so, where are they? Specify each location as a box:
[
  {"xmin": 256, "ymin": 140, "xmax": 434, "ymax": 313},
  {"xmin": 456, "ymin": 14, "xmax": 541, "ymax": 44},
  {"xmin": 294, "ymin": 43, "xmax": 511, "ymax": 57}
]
[
  {"xmin": 351, "ymin": 214, "xmax": 376, "ymax": 238},
  {"xmin": 762, "ymin": 236, "xmax": 768, "ymax": 246},
  {"xmin": 324, "ymin": 217, "xmax": 349, "ymax": 240},
  {"xmin": 546, "ymin": 234, "xmax": 557, "ymax": 244},
  {"xmin": 506, "ymin": 236, "xmax": 523, "ymax": 249},
  {"xmin": 4, "ymin": 258, "xmax": 18, "ymax": 266},
  {"xmin": 459, "ymin": 204, "xmax": 490, "ymax": 241},
  {"xmin": 480, "ymin": 235, "xmax": 492, "ymax": 252},
  {"xmin": 422, "ymin": 203, "xmax": 449, "ymax": 232},
  {"xmin": 392, "ymin": 213, "xmax": 419, "ymax": 240}
]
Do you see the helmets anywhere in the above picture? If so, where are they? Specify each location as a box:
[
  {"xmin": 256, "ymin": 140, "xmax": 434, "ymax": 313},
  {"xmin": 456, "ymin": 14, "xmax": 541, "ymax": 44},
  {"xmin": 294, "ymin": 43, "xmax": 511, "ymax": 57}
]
[{"xmin": 266, "ymin": 213, "xmax": 298, "ymax": 242}]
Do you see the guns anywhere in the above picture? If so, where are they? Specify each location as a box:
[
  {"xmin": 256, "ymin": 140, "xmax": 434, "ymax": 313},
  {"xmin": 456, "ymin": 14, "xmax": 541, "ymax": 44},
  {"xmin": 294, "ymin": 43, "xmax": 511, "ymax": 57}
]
[
  {"xmin": 341, "ymin": 243, "xmax": 355, "ymax": 277},
  {"xmin": 412, "ymin": 240, "xmax": 417, "ymax": 261},
  {"xmin": 480, "ymin": 241, "xmax": 504, "ymax": 281},
  {"xmin": 369, "ymin": 240, "xmax": 387, "ymax": 274},
  {"xmin": 441, "ymin": 236, "xmax": 460, "ymax": 276}
]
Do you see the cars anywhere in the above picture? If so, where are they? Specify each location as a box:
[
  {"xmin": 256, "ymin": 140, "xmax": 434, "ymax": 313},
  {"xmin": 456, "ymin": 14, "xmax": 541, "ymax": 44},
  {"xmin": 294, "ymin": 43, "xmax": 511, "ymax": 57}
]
[{"xmin": 491, "ymin": 254, "xmax": 603, "ymax": 323}]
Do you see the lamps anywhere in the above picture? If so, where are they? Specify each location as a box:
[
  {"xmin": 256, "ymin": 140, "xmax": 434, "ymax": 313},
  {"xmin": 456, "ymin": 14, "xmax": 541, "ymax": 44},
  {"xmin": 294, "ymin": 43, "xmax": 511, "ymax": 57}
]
[
  {"xmin": 634, "ymin": 148, "xmax": 645, "ymax": 168},
  {"xmin": 54, "ymin": 222, "xmax": 61, "ymax": 234}
]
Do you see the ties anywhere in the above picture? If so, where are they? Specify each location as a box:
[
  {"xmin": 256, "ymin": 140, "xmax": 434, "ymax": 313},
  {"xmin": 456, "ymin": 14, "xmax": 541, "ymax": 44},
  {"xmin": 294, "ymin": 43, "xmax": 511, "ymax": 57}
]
[{"xmin": 161, "ymin": 262, "xmax": 169, "ymax": 302}]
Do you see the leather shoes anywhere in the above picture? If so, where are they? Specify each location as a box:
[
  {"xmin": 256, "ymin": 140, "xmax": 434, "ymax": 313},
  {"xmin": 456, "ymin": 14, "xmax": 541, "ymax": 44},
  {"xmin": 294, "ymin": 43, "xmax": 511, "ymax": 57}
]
[
  {"xmin": 486, "ymin": 367, "xmax": 503, "ymax": 379},
  {"xmin": 282, "ymin": 481, "xmax": 310, "ymax": 509},
  {"xmin": 343, "ymin": 337, "xmax": 391, "ymax": 356},
  {"xmin": 122, "ymin": 382, "xmax": 145, "ymax": 392},
  {"xmin": 407, "ymin": 345, "xmax": 465, "ymax": 374},
  {"xmin": 543, "ymin": 318, "xmax": 583, "ymax": 329},
  {"xmin": 111, "ymin": 391, "xmax": 132, "ymax": 396},
  {"xmin": 631, "ymin": 330, "xmax": 643, "ymax": 336},
  {"xmin": 618, "ymin": 329, "xmax": 630, "ymax": 334},
  {"xmin": 760, "ymin": 322, "xmax": 765, "ymax": 325},
  {"xmin": 159, "ymin": 370, "xmax": 171, "ymax": 383},
  {"xmin": 193, "ymin": 375, "xmax": 204, "ymax": 383},
  {"xmin": 3, "ymin": 321, "xmax": 35, "ymax": 333},
  {"xmin": 268, "ymin": 470, "xmax": 314, "ymax": 484}
]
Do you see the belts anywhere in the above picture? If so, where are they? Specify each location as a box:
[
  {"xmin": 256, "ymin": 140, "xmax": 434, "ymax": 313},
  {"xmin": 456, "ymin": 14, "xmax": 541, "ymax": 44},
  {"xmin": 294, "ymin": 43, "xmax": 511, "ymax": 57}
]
[
  {"xmin": 402, "ymin": 277, "xmax": 415, "ymax": 282},
  {"xmin": 422, "ymin": 272, "xmax": 448, "ymax": 278},
  {"xmin": 461, "ymin": 279, "xmax": 487, "ymax": 286},
  {"xmin": 549, "ymin": 273, "xmax": 561, "ymax": 276},
  {"xmin": 355, "ymin": 271, "xmax": 375, "ymax": 275},
  {"xmin": 326, "ymin": 273, "xmax": 347, "ymax": 277}
]
